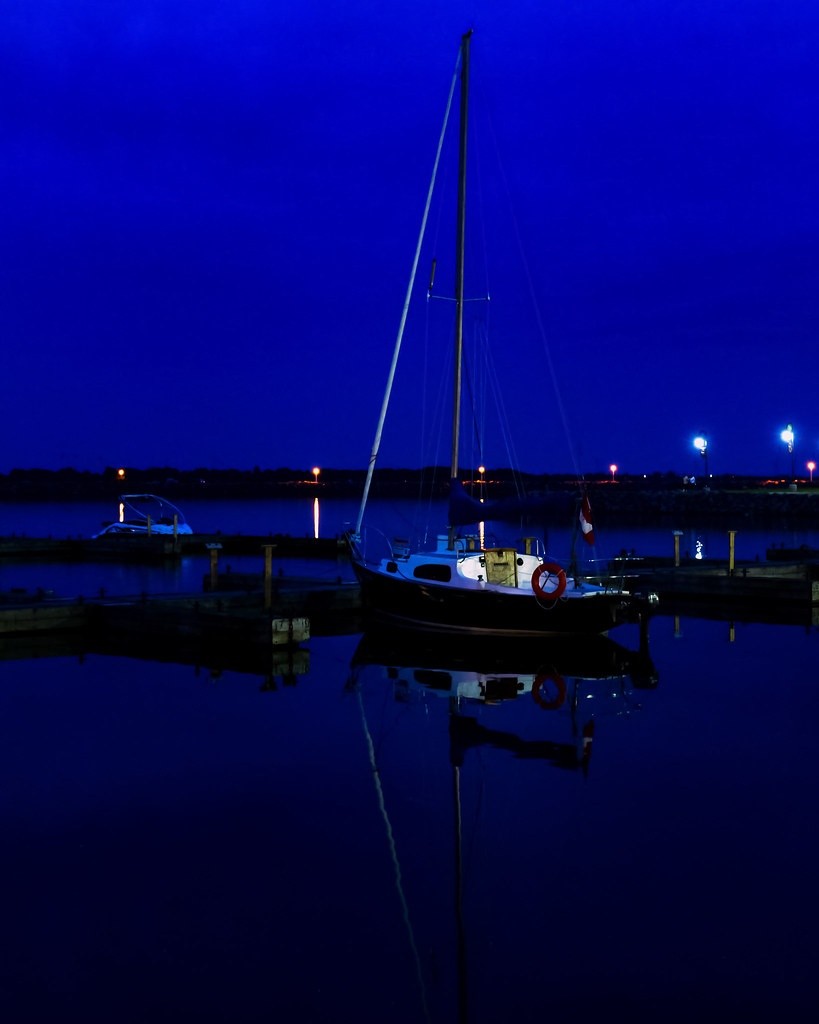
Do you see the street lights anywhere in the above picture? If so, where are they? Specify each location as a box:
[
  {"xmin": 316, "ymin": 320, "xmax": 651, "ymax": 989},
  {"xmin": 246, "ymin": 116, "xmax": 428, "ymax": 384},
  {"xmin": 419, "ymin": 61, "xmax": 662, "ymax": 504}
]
[
  {"xmin": 312, "ymin": 467, "xmax": 320, "ymax": 482},
  {"xmin": 784, "ymin": 425, "xmax": 796, "ymax": 476},
  {"xmin": 692, "ymin": 435, "xmax": 709, "ymax": 477},
  {"xmin": 608, "ymin": 464, "xmax": 618, "ymax": 483},
  {"xmin": 807, "ymin": 462, "xmax": 815, "ymax": 481}
]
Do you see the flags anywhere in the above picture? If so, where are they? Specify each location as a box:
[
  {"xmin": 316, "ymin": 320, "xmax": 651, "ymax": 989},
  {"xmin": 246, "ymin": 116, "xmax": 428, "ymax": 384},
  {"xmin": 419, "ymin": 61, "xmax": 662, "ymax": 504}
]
[
  {"xmin": 578, "ymin": 494, "xmax": 596, "ymax": 544},
  {"xmin": 581, "ymin": 716, "xmax": 595, "ymax": 777}
]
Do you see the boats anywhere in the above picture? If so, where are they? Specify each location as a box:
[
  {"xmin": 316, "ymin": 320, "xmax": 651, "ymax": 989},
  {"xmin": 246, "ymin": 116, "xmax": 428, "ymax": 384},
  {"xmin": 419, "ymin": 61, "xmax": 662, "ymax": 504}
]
[{"xmin": 92, "ymin": 493, "xmax": 193, "ymax": 540}]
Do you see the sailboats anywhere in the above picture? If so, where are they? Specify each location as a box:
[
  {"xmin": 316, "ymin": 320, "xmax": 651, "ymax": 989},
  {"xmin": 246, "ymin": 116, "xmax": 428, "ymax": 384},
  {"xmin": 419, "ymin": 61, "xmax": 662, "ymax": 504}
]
[
  {"xmin": 345, "ymin": 631, "xmax": 656, "ymax": 1023},
  {"xmin": 347, "ymin": 31, "xmax": 662, "ymax": 640}
]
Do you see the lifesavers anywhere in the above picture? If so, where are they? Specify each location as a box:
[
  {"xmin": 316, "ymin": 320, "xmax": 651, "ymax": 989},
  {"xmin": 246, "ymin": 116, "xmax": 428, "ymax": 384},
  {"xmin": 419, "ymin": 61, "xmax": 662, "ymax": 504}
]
[
  {"xmin": 530, "ymin": 673, "xmax": 570, "ymax": 713},
  {"xmin": 532, "ymin": 563, "xmax": 567, "ymax": 599}
]
